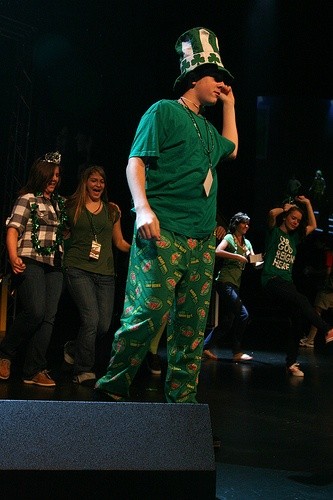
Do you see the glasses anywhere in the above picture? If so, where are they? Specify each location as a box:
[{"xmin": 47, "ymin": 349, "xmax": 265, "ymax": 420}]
[{"xmin": 202, "ymin": 72, "xmax": 233, "ymax": 86}]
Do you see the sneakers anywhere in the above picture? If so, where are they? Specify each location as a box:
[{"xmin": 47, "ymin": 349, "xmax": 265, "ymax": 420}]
[
  {"xmin": 299, "ymin": 338, "xmax": 314, "ymax": 347},
  {"xmin": 145, "ymin": 351, "xmax": 162, "ymax": 374},
  {"xmin": 325, "ymin": 328, "xmax": 333, "ymax": 343},
  {"xmin": 64, "ymin": 340, "xmax": 76, "ymax": 365},
  {"xmin": 23, "ymin": 369, "xmax": 56, "ymax": 386},
  {"xmin": 289, "ymin": 363, "xmax": 305, "ymax": 376},
  {"xmin": 72, "ymin": 372, "xmax": 98, "ymax": 384},
  {"xmin": 0, "ymin": 358, "xmax": 11, "ymax": 379}
]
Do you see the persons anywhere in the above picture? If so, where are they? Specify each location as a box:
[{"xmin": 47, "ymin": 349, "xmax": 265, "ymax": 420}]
[
  {"xmin": 0, "ymin": 152, "xmax": 67, "ymax": 386},
  {"xmin": 63, "ymin": 26, "xmax": 333, "ymax": 403}
]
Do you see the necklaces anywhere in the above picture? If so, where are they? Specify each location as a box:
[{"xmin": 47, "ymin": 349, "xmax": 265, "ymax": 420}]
[
  {"xmin": 180, "ymin": 97, "xmax": 200, "ymax": 108},
  {"xmin": 93, "ymin": 200, "xmax": 101, "ymax": 213}
]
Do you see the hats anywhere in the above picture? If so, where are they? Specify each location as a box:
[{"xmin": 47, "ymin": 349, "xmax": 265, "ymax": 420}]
[{"xmin": 172, "ymin": 27, "xmax": 235, "ymax": 94}]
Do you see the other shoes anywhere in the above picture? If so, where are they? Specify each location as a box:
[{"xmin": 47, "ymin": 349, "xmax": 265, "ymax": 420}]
[
  {"xmin": 93, "ymin": 384, "xmax": 126, "ymax": 402},
  {"xmin": 213, "ymin": 436, "xmax": 222, "ymax": 447}
]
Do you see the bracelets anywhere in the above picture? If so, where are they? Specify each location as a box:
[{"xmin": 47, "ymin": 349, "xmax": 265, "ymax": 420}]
[{"xmin": 282, "ymin": 207, "xmax": 285, "ymax": 213}]
